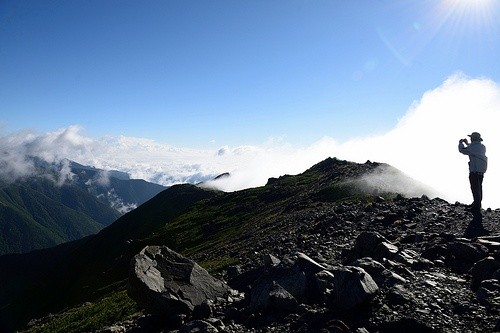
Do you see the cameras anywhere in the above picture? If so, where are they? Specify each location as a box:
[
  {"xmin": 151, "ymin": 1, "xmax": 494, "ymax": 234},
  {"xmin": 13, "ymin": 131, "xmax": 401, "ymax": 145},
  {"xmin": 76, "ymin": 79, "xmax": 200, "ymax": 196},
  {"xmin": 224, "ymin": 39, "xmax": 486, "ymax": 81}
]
[{"xmin": 461, "ymin": 139, "xmax": 466, "ymax": 142}]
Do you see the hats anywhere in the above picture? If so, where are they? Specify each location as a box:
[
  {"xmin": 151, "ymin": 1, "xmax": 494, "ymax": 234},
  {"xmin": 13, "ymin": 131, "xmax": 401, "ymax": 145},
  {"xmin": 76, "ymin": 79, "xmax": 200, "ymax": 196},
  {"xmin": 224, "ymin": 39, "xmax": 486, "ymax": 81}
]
[{"xmin": 467, "ymin": 132, "xmax": 483, "ymax": 141}]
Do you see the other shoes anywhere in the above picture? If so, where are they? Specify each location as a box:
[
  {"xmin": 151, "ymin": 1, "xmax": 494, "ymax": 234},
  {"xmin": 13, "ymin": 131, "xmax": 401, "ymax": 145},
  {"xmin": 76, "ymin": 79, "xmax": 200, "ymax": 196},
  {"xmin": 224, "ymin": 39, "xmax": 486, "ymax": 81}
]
[{"xmin": 465, "ymin": 204, "xmax": 480, "ymax": 211}]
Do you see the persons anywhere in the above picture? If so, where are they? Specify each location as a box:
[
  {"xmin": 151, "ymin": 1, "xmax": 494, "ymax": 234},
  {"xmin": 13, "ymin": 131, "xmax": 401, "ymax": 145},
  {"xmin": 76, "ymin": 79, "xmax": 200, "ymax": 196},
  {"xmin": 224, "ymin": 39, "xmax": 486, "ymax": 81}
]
[{"xmin": 458, "ymin": 132, "xmax": 487, "ymax": 212}]
